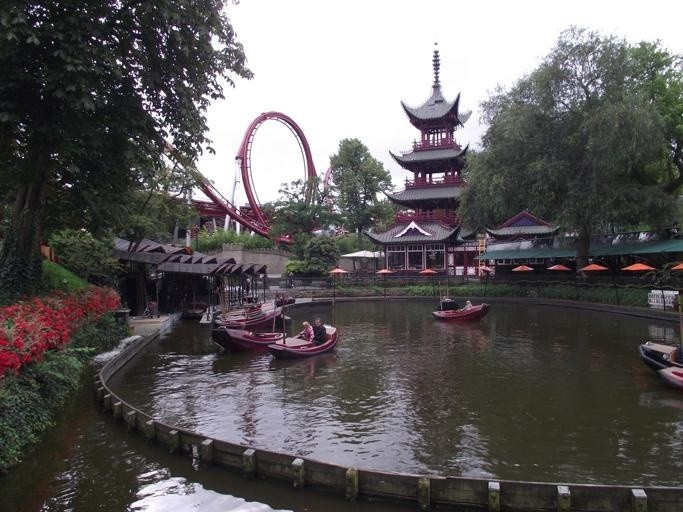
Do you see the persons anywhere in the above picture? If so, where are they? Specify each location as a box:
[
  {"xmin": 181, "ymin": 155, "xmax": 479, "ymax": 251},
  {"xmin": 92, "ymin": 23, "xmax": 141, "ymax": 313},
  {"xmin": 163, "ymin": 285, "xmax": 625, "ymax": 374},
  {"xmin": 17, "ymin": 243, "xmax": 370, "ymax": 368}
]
[
  {"xmin": 300, "ymin": 321, "xmax": 314, "ymax": 342},
  {"xmin": 312, "ymin": 318, "xmax": 326, "ymax": 345},
  {"xmin": 460, "ymin": 301, "xmax": 472, "ymax": 312}
]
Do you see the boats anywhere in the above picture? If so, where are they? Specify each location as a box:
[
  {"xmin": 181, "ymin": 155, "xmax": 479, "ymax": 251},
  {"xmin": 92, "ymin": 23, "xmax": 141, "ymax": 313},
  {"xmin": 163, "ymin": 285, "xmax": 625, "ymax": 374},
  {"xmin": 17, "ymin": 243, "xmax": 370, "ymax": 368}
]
[
  {"xmin": 638, "ymin": 341, "xmax": 683, "ymax": 390},
  {"xmin": 212, "ymin": 303, "xmax": 338, "ymax": 358},
  {"xmin": 432, "ymin": 303, "xmax": 491, "ymax": 323}
]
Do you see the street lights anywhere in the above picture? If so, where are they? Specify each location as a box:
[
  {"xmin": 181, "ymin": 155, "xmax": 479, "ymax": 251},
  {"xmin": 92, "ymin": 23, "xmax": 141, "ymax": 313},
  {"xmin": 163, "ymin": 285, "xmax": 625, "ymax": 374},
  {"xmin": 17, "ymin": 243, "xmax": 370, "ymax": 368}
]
[{"xmin": 246, "ymin": 271, "xmax": 264, "ymax": 303}]
